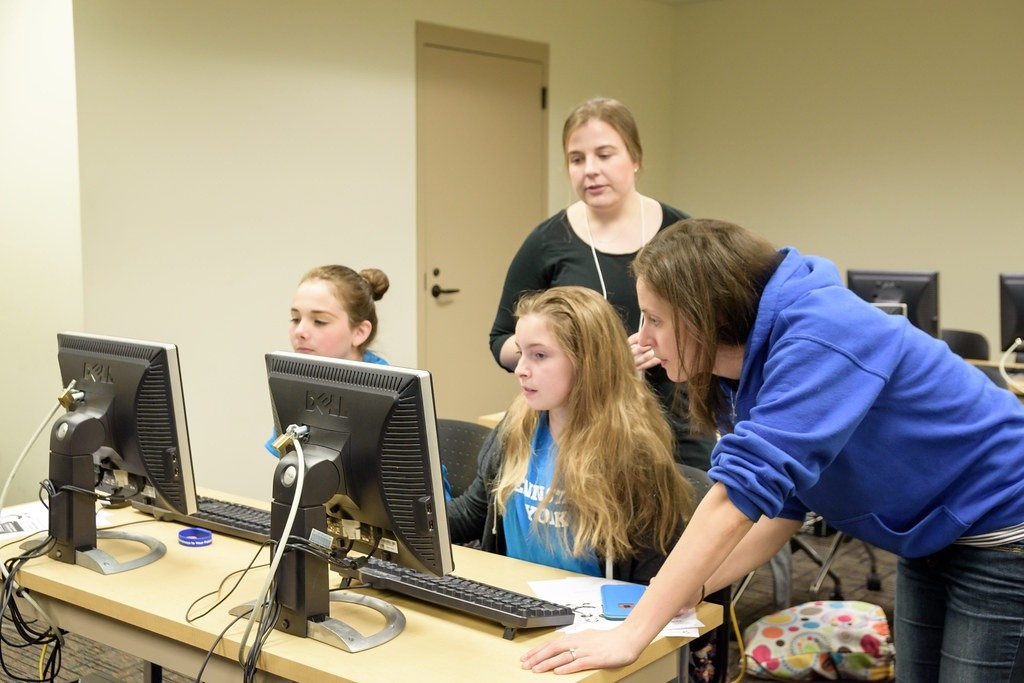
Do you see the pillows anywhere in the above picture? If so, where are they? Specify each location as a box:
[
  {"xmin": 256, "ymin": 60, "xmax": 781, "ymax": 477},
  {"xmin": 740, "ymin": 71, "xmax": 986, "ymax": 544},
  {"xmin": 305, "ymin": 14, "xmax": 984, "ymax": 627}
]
[{"xmin": 739, "ymin": 600, "xmax": 896, "ymax": 681}]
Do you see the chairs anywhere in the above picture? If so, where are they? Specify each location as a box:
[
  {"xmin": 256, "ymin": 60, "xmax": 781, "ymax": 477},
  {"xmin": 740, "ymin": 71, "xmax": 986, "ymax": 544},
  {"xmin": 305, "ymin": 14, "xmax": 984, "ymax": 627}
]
[
  {"xmin": 597, "ymin": 463, "xmax": 730, "ymax": 683},
  {"xmin": 941, "ymin": 329, "xmax": 989, "ymax": 361},
  {"xmin": 436, "ymin": 419, "xmax": 495, "ymax": 499}
]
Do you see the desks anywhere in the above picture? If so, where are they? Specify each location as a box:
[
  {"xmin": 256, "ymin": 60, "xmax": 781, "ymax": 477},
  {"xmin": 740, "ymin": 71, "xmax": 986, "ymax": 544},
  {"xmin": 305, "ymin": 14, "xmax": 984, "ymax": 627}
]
[{"xmin": 0, "ymin": 489, "xmax": 723, "ymax": 683}]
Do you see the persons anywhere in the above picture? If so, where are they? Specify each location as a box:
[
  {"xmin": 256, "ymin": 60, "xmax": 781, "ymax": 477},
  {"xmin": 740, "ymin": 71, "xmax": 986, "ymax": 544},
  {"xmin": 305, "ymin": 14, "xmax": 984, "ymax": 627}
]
[
  {"xmin": 520, "ymin": 218, "xmax": 1024, "ymax": 683},
  {"xmin": 262, "ymin": 266, "xmax": 453, "ymax": 504},
  {"xmin": 445, "ymin": 286, "xmax": 694, "ymax": 585},
  {"xmin": 489, "ymin": 98, "xmax": 718, "ymax": 470}
]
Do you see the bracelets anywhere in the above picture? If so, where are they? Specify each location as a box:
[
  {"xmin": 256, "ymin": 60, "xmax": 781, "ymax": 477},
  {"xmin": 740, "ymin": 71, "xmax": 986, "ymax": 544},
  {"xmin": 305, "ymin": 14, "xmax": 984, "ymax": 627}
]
[
  {"xmin": 698, "ymin": 584, "xmax": 705, "ymax": 605},
  {"xmin": 178, "ymin": 529, "xmax": 213, "ymax": 547}
]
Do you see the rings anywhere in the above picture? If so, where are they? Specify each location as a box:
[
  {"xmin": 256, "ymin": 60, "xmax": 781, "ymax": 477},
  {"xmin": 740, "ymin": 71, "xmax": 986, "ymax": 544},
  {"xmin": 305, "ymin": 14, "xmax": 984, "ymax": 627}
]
[{"xmin": 570, "ymin": 649, "xmax": 576, "ymax": 661}]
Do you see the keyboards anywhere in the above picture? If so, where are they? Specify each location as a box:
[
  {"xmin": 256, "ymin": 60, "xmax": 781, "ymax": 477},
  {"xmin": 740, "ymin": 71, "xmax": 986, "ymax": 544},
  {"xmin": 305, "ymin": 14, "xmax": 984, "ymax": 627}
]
[
  {"xmin": 131, "ymin": 491, "xmax": 274, "ymax": 547},
  {"xmin": 326, "ymin": 555, "xmax": 575, "ymax": 641}
]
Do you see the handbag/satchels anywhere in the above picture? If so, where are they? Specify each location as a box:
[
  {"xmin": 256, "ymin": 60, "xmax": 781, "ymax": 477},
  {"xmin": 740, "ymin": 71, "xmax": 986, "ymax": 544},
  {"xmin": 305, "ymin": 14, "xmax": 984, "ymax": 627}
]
[{"xmin": 738, "ymin": 600, "xmax": 896, "ymax": 681}]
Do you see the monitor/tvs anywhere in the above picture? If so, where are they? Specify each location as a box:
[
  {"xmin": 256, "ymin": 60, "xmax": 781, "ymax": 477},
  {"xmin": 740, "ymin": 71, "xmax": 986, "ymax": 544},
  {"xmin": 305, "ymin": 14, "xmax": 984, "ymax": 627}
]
[
  {"xmin": 227, "ymin": 349, "xmax": 457, "ymax": 653},
  {"xmin": 19, "ymin": 332, "xmax": 199, "ymax": 575},
  {"xmin": 847, "ymin": 270, "xmax": 943, "ymax": 340},
  {"xmin": 999, "ymin": 273, "xmax": 1024, "ymax": 364}
]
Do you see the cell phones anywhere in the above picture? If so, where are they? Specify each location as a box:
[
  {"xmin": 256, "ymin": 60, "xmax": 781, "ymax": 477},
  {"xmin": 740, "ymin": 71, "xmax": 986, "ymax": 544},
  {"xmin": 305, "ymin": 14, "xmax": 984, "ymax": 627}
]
[{"xmin": 600, "ymin": 584, "xmax": 646, "ymax": 621}]
